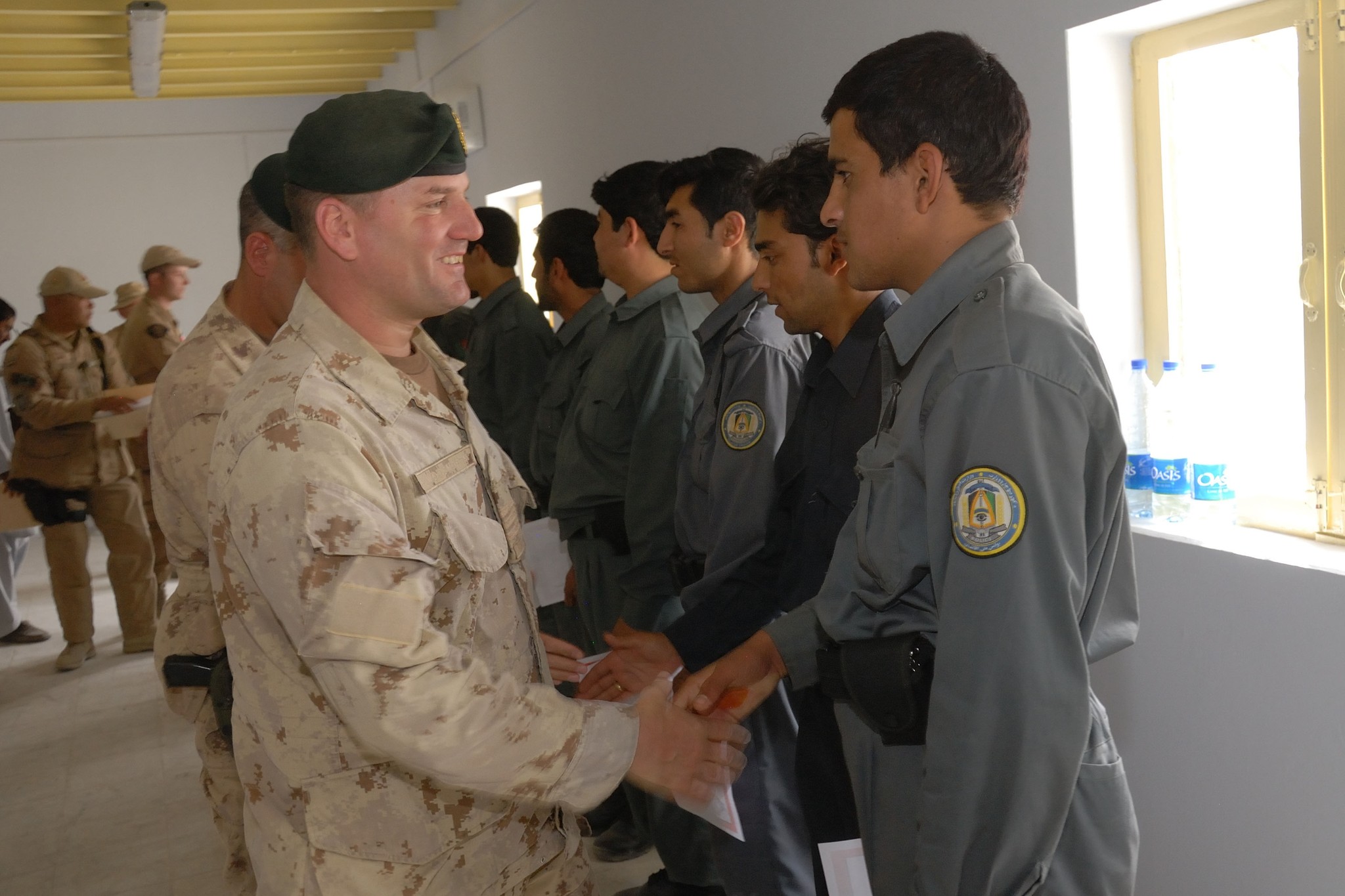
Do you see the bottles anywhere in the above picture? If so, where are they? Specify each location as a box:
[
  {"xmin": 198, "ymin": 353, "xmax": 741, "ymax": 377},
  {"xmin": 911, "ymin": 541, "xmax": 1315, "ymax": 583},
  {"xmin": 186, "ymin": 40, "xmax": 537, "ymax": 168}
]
[
  {"xmin": 1119, "ymin": 359, "xmax": 1156, "ymax": 518},
  {"xmin": 1187, "ymin": 364, "xmax": 1237, "ymax": 532},
  {"xmin": 1147, "ymin": 361, "xmax": 1189, "ymax": 527}
]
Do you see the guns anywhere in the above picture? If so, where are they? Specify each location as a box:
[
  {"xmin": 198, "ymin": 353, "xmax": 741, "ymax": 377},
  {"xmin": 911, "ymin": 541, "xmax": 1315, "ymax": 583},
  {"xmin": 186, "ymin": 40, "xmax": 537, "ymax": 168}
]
[{"xmin": 161, "ymin": 655, "xmax": 216, "ymax": 689}]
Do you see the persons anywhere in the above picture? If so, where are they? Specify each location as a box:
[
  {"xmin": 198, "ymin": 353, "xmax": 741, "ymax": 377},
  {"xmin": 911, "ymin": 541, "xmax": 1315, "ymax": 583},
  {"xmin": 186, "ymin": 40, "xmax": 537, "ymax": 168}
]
[{"xmin": 0, "ymin": 30, "xmax": 1144, "ymax": 896}]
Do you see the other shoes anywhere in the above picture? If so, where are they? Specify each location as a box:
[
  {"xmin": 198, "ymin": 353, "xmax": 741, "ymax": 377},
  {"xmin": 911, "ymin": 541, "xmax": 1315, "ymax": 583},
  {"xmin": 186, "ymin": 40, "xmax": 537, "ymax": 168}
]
[
  {"xmin": 0, "ymin": 620, "xmax": 50, "ymax": 644},
  {"xmin": 123, "ymin": 631, "xmax": 155, "ymax": 655},
  {"xmin": 580, "ymin": 794, "xmax": 654, "ymax": 863},
  {"xmin": 614, "ymin": 867, "xmax": 725, "ymax": 896},
  {"xmin": 55, "ymin": 636, "xmax": 97, "ymax": 670}
]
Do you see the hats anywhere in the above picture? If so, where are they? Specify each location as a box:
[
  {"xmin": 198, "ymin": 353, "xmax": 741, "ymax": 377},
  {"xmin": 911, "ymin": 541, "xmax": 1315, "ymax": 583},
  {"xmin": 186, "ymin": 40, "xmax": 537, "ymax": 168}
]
[
  {"xmin": 282, "ymin": 88, "xmax": 468, "ymax": 195},
  {"xmin": 38, "ymin": 267, "xmax": 109, "ymax": 300},
  {"xmin": 142, "ymin": 244, "xmax": 203, "ymax": 273},
  {"xmin": 248, "ymin": 152, "xmax": 298, "ymax": 236},
  {"xmin": 110, "ymin": 280, "xmax": 149, "ymax": 314}
]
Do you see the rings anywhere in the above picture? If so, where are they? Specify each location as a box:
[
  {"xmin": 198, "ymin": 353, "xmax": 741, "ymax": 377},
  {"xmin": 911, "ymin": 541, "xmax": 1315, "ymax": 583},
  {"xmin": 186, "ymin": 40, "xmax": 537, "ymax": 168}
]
[{"xmin": 615, "ymin": 680, "xmax": 623, "ymax": 692}]
